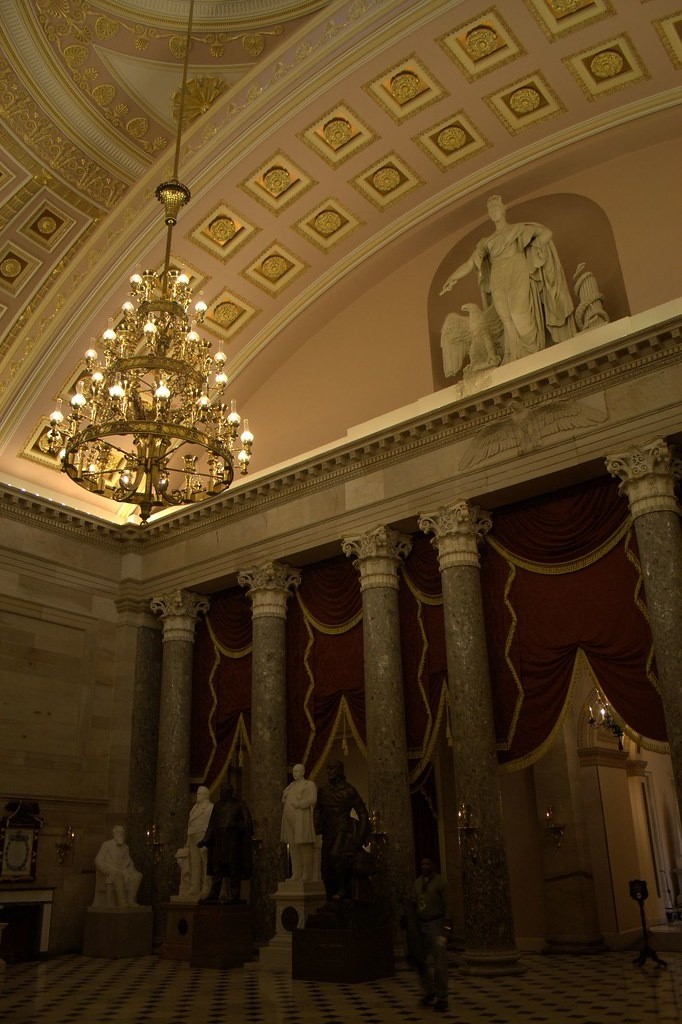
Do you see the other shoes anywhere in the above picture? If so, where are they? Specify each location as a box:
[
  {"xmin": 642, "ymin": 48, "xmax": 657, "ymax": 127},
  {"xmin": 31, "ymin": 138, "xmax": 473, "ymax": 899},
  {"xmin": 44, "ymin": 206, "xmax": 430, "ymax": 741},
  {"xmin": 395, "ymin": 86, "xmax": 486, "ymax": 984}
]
[
  {"xmin": 432, "ymin": 1000, "xmax": 449, "ymax": 1012},
  {"xmin": 417, "ymin": 993, "xmax": 434, "ymax": 1009}
]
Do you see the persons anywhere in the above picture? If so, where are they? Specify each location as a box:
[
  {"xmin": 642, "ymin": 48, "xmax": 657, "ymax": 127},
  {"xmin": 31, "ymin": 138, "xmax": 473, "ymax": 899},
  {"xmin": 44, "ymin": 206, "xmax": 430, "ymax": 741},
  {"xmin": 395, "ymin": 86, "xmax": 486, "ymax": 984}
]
[
  {"xmin": 442, "ymin": 194, "xmax": 576, "ymax": 368},
  {"xmin": 315, "ymin": 759, "xmax": 369, "ymax": 911},
  {"xmin": 403, "ymin": 851, "xmax": 454, "ymax": 1011},
  {"xmin": 95, "ymin": 824, "xmax": 143, "ymax": 908},
  {"xmin": 280, "ymin": 763, "xmax": 318, "ymax": 880},
  {"xmin": 197, "ymin": 782, "xmax": 254, "ymax": 899},
  {"xmin": 185, "ymin": 786, "xmax": 214, "ymax": 895}
]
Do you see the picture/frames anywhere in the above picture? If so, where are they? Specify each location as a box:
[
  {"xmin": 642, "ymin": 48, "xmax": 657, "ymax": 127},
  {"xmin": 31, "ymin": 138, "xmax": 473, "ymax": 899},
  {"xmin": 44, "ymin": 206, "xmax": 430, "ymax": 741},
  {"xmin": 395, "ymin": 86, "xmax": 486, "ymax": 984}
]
[{"xmin": 0, "ymin": 815, "xmax": 46, "ymax": 884}]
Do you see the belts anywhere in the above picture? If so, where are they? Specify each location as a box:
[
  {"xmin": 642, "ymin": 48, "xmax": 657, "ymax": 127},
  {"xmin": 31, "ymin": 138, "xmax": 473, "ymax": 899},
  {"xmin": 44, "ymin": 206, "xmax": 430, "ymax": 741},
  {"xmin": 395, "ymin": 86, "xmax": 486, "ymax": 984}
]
[{"xmin": 416, "ymin": 912, "xmax": 446, "ymax": 923}]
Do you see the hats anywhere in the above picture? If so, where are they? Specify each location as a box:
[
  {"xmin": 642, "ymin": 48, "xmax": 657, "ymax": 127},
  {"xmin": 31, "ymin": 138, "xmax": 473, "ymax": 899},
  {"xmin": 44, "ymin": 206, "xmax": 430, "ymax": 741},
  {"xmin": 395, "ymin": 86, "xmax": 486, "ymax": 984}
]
[{"xmin": 420, "ymin": 850, "xmax": 438, "ymax": 865}]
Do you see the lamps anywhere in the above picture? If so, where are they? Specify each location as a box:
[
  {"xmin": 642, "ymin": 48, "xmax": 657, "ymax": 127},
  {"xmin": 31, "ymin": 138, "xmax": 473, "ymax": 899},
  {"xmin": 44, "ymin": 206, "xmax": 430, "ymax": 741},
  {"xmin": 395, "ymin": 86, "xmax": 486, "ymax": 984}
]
[
  {"xmin": 544, "ymin": 804, "xmax": 567, "ymax": 858},
  {"xmin": 147, "ymin": 817, "xmax": 163, "ymax": 853},
  {"xmin": 458, "ymin": 801, "xmax": 479, "ymax": 830},
  {"xmin": 56, "ymin": 825, "xmax": 74, "ymax": 852},
  {"xmin": 370, "ymin": 809, "xmax": 388, "ymax": 841},
  {"xmin": 582, "ymin": 662, "xmax": 624, "ymax": 750},
  {"xmin": 48, "ymin": 0, "xmax": 254, "ymax": 528}
]
